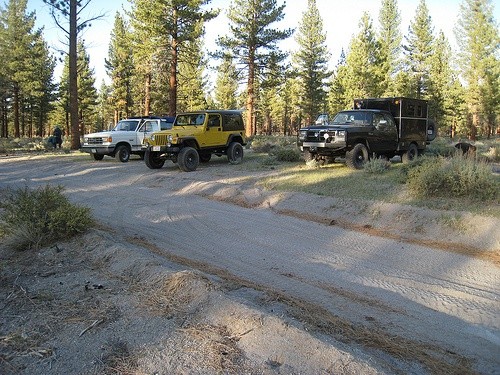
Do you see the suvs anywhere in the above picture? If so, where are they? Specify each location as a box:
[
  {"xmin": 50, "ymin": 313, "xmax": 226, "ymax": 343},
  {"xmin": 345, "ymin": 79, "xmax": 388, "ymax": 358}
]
[
  {"xmin": 138, "ymin": 110, "xmax": 247, "ymax": 172},
  {"xmin": 297, "ymin": 97, "xmax": 428, "ymax": 170},
  {"xmin": 79, "ymin": 116, "xmax": 175, "ymax": 162}
]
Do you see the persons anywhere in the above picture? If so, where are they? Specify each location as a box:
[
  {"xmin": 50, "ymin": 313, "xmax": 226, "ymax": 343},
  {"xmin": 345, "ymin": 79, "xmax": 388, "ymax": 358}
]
[
  {"xmin": 455, "ymin": 143, "xmax": 476, "ymax": 155},
  {"xmin": 53, "ymin": 126, "xmax": 62, "ymax": 148}
]
